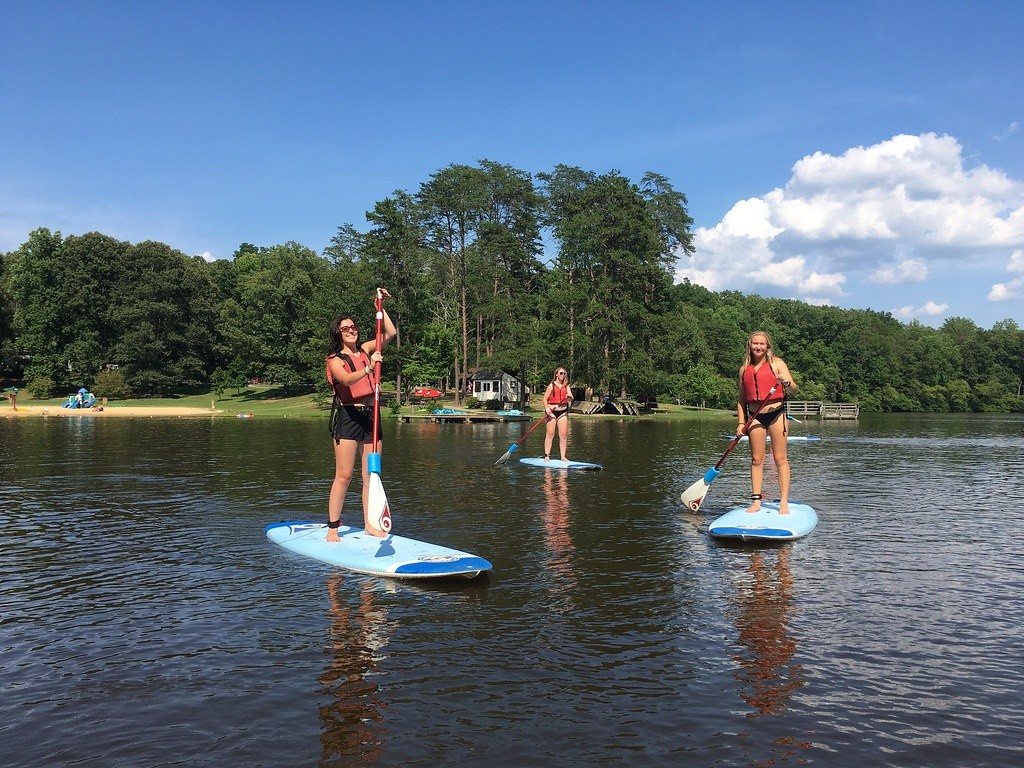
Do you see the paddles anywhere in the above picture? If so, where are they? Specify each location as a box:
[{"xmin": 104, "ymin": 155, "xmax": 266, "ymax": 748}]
[
  {"xmin": 368, "ymin": 287, "xmax": 393, "ymax": 532},
  {"xmin": 494, "ymin": 399, "xmax": 570, "ymax": 465},
  {"xmin": 679, "ymin": 376, "xmax": 785, "ymax": 514}
]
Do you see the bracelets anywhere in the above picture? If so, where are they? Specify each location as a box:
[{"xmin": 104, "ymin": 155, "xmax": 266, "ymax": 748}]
[
  {"xmin": 785, "ymin": 381, "xmax": 791, "ymax": 387},
  {"xmin": 738, "ymin": 422, "xmax": 745, "ymax": 425},
  {"xmin": 546, "ymin": 408, "xmax": 549, "ymax": 412},
  {"xmin": 365, "ymin": 366, "xmax": 374, "ymax": 374}
]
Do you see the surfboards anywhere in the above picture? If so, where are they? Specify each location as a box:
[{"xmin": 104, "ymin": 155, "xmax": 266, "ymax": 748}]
[
  {"xmin": 706, "ymin": 497, "xmax": 819, "ymax": 541},
  {"xmin": 264, "ymin": 522, "xmax": 493, "ymax": 579},
  {"xmin": 520, "ymin": 457, "xmax": 603, "ymax": 471}
]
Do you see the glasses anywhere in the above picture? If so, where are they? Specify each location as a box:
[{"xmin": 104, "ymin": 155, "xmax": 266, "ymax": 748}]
[
  {"xmin": 560, "ymin": 372, "xmax": 566, "ymax": 375},
  {"xmin": 340, "ymin": 325, "xmax": 356, "ymax": 333}
]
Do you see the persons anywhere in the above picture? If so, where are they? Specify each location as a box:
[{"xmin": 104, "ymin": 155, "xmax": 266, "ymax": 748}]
[
  {"xmin": 90, "ymin": 405, "xmax": 103, "ymax": 412},
  {"xmin": 543, "ymin": 367, "xmax": 574, "ymax": 461},
  {"xmin": 326, "ymin": 289, "xmax": 397, "ymax": 543},
  {"xmin": 736, "ymin": 331, "xmax": 798, "ymax": 515},
  {"xmin": 8, "ymin": 394, "xmax": 16, "ymax": 404}
]
